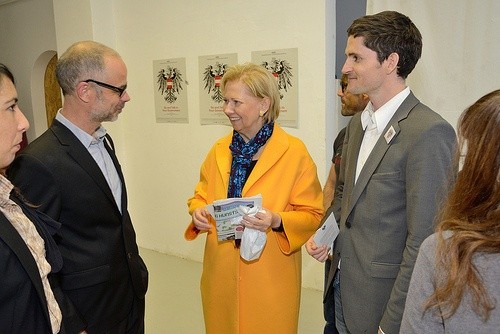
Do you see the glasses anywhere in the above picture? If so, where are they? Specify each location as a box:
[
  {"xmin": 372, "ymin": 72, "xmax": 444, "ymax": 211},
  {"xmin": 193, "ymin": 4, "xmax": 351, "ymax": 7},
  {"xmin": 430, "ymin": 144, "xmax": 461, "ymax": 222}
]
[
  {"xmin": 341, "ymin": 81, "xmax": 348, "ymax": 93},
  {"xmin": 85, "ymin": 79, "xmax": 127, "ymax": 97}
]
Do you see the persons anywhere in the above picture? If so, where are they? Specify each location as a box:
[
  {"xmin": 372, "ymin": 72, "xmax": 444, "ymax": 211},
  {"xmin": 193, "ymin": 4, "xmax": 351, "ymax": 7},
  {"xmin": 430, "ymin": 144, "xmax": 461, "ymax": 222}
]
[
  {"xmin": 305, "ymin": 10, "xmax": 459, "ymax": 334},
  {"xmin": 0, "ymin": 63, "xmax": 88, "ymax": 334},
  {"xmin": 185, "ymin": 63, "xmax": 324, "ymax": 334},
  {"xmin": 323, "ymin": 74, "xmax": 370, "ymax": 334},
  {"xmin": 399, "ymin": 89, "xmax": 500, "ymax": 334},
  {"xmin": 5, "ymin": 41, "xmax": 148, "ymax": 334}
]
